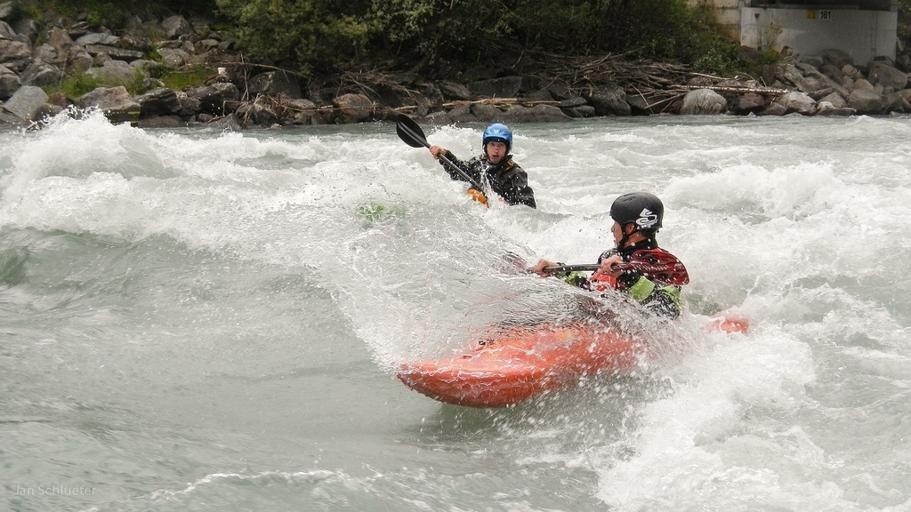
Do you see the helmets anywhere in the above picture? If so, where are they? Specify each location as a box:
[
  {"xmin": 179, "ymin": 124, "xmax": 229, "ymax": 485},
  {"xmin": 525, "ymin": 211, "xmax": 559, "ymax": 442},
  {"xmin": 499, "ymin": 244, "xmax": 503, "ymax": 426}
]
[
  {"xmin": 481, "ymin": 122, "xmax": 514, "ymax": 151},
  {"xmin": 610, "ymin": 190, "xmax": 664, "ymax": 231}
]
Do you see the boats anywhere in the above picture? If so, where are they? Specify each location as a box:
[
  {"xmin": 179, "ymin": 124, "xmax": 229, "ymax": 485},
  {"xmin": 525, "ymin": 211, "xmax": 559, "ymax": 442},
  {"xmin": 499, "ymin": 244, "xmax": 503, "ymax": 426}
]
[{"xmin": 396, "ymin": 316, "xmax": 749, "ymax": 407}]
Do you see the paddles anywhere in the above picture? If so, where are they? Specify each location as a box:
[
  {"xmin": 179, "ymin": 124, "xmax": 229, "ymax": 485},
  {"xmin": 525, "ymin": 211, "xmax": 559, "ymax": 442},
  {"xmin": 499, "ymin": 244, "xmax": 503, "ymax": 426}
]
[{"xmin": 396, "ymin": 113, "xmax": 487, "ymax": 195}]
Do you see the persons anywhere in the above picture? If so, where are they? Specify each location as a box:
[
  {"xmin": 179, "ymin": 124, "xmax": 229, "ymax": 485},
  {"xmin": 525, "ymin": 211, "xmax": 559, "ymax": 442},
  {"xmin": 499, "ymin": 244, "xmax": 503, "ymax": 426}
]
[
  {"xmin": 430, "ymin": 121, "xmax": 538, "ymax": 221},
  {"xmin": 532, "ymin": 190, "xmax": 691, "ymax": 333}
]
